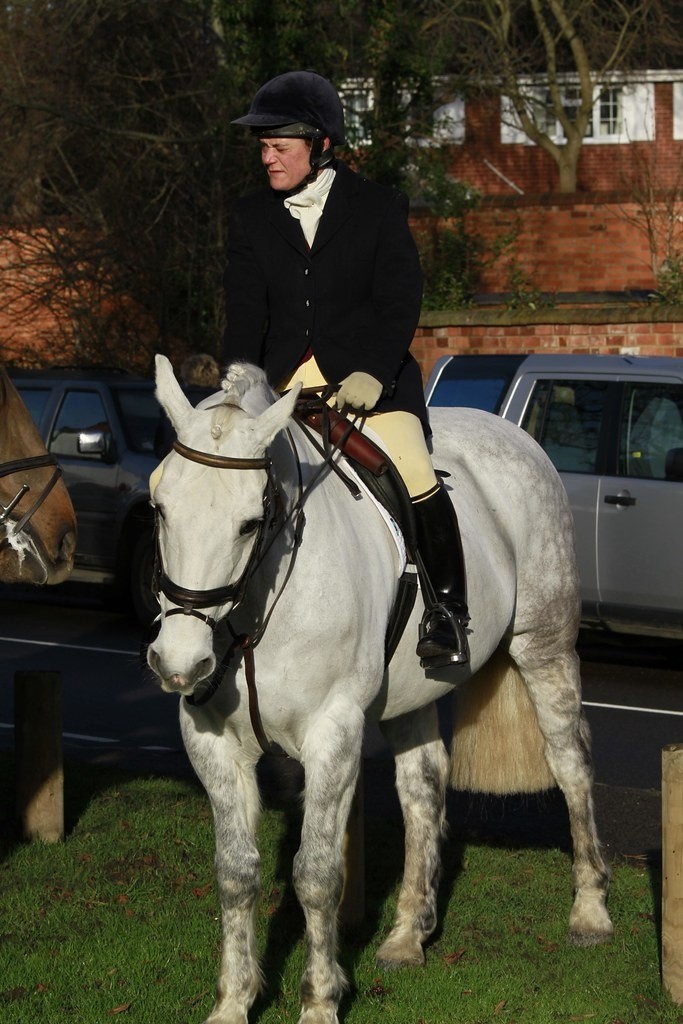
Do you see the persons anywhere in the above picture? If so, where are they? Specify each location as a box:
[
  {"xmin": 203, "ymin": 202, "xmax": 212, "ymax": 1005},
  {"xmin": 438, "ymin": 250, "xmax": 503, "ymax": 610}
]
[{"xmin": 148, "ymin": 71, "xmax": 470, "ymax": 664}]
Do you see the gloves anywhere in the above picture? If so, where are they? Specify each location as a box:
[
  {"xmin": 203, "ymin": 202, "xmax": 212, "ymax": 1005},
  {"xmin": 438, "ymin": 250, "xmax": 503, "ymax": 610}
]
[{"xmin": 331, "ymin": 371, "xmax": 384, "ymax": 411}]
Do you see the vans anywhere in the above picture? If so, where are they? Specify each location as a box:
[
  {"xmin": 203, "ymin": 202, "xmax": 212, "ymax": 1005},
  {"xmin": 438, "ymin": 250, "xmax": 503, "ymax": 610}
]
[{"xmin": 424, "ymin": 354, "xmax": 683, "ymax": 643}]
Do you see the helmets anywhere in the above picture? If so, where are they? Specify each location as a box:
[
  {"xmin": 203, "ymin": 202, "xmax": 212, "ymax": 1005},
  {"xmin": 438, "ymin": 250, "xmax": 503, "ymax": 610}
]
[{"xmin": 229, "ymin": 71, "xmax": 346, "ymax": 168}]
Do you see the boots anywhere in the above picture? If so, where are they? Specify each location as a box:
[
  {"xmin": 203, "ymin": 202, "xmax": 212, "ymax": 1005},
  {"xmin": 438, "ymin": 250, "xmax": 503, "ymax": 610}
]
[{"xmin": 410, "ymin": 475, "xmax": 470, "ymax": 665}]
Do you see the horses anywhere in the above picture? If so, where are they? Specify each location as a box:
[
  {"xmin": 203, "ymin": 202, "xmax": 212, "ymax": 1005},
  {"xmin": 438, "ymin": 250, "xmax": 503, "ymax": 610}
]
[
  {"xmin": 144, "ymin": 354, "xmax": 616, "ymax": 1024},
  {"xmin": 0, "ymin": 356, "xmax": 81, "ymax": 586}
]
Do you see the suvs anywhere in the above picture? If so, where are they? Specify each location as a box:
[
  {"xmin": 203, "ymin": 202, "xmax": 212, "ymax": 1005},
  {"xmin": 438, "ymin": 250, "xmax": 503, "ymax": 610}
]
[{"xmin": 1, "ymin": 364, "xmax": 219, "ymax": 631}]
